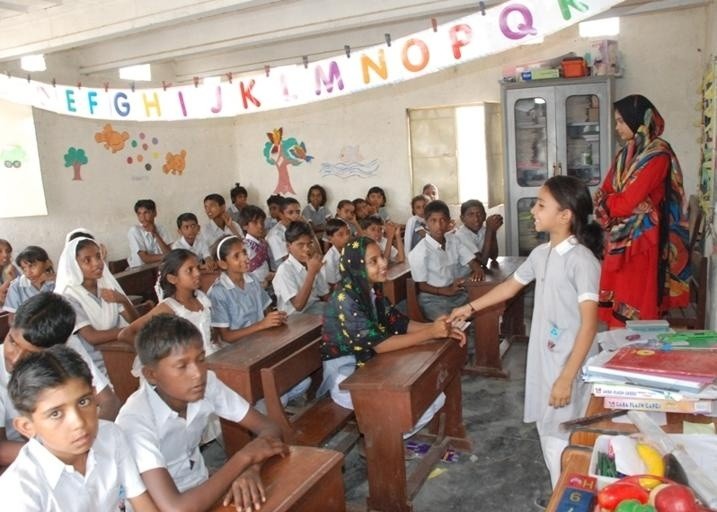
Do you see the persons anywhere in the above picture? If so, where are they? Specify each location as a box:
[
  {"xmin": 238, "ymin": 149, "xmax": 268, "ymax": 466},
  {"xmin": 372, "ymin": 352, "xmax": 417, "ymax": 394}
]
[
  {"xmin": 593, "ymin": 94, "xmax": 691, "ymax": 331},
  {"xmin": 445, "ymin": 175, "xmax": 605, "ymax": 509},
  {"xmin": 1, "ymin": 183, "xmax": 505, "ymax": 512}
]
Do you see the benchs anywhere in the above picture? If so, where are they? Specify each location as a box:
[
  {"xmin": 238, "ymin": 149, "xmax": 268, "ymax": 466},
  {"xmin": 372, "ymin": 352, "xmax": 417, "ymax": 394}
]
[{"xmin": 257, "ymin": 334, "xmax": 362, "ymax": 456}]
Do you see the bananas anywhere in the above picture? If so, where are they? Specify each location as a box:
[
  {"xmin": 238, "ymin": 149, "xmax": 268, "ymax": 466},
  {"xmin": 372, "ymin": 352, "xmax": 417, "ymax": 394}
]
[{"xmin": 637, "ymin": 445, "xmax": 665, "ymax": 485}]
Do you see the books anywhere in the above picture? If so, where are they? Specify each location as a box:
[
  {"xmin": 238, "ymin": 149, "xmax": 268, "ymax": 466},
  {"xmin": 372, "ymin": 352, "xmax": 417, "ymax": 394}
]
[
  {"xmin": 515, "ymin": 94, "xmax": 599, "ymax": 256},
  {"xmin": 581, "ymin": 328, "xmax": 717, "ymax": 418}
]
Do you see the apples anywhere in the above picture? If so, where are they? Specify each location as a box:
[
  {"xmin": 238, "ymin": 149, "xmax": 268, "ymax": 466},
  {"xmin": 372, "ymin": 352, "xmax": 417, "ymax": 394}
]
[{"xmin": 614, "ymin": 498, "xmax": 654, "ymax": 512}]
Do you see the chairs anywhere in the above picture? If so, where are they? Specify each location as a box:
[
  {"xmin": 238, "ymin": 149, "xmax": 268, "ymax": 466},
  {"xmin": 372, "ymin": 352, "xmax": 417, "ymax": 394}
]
[
  {"xmin": 655, "ymin": 245, "xmax": 708, "ymax": 332},
  {"xmin": 676, "ymin": 193, "xmax": 703, "ymax": 252},
  {"xmin": 109, "ymin": 259, "xmax": 128, "ymax": 274}
]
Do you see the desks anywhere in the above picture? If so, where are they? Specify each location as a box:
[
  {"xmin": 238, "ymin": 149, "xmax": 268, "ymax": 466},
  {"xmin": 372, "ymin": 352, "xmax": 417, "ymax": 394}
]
[
  {"xmin": 375, "ymin": 253, "xmax": 413, "ymax": 306},
  {"xmin": 90, "ymin": 332, "xmax": 144, "ymax": 407},
  {"xmin": 108, "ymin": 263, "xmax": 158, "ymax": 305},
  {"xmin": 571, "ymin": 374, "xmax": 717, "ymax": 448},
  {"xmin": 337, "ymin": 319, "xmax": 476, "ymax": 511},
  {"xmin": 459, "ymin": 255, "xmax": 532, "ymax": 380},
  {"xmin": 547, "ymin": 444, "xmax": 717, "ymax": 512},
  {"xmin": 195, "ymin": 310, "xmax": 323, "ymax": 409},
  {"xmin": 194, "ymin": 446, "xmax": 346, "ymax": 512}
]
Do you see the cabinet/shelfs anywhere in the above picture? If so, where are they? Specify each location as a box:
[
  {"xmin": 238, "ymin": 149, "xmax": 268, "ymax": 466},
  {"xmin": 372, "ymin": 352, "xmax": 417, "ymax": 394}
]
[{"xmin": 497, "ymin": 74, "xmax": 613, "ymax": 257}]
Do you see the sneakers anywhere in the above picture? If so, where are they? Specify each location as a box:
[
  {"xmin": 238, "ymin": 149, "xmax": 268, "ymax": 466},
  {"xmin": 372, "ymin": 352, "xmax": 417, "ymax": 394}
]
[
  {"xmin": 530, "ymin": 490, "xmax": 559, "ymax": 512},
  {"xmin": 355, "ymin": 434, "xmax": 422, "ymax": 462}
]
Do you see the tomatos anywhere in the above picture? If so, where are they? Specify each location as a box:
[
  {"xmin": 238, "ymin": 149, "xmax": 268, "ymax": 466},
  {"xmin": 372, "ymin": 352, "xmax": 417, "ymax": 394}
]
[
  {"xmin": 653, "ymin": 486, "xmax": 695, "ymax": 512},
  {"xmin": 591, "ymin": 486, "xmax": 650, "ymax": 509}
]
